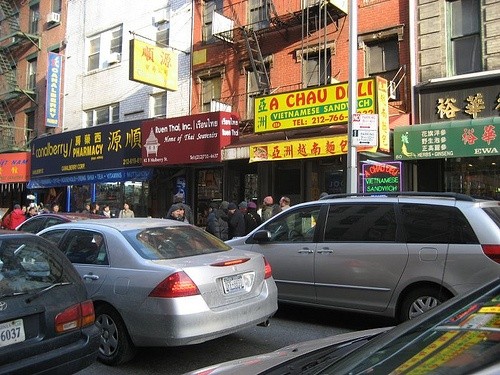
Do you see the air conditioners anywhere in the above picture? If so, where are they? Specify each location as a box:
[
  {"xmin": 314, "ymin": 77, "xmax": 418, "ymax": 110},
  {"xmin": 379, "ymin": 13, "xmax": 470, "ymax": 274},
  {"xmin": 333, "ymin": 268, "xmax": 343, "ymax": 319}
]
[
  {"xmin": 107, "ymin": 52, "xmax": 121, "ymax": 63},
  {"xmin": 47, "ymin": 12, "xmax": 60, "ymax": 24}
]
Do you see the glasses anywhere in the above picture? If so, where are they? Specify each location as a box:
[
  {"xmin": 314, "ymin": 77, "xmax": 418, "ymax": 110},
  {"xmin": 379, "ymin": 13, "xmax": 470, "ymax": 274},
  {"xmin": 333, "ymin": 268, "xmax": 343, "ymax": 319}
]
[{"xmin": 180, "ymin": 209, "xmax": 185, "ymax": 210}]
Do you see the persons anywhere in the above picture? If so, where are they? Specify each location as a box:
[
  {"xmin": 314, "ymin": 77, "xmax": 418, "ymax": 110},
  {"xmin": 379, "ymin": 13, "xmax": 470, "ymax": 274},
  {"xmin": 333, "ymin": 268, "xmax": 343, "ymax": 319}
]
[
  {"xmin": 80, "ymin": 203, "xmax": 90, "ymax": 213},
  {"xmin": 100, "ymin": 204, "xmax": 112, "ymax": 218},
  {"xmin": 166, "ymin": 203, "xmax": 191, "ymax": 225},
  {"xmin": 313, "ymin": 192, "xmax": 328, "ymax": 223},
  {"xmin": 118, "ymin": 202, "xmax": 134, "ymax": 218},
  {"xmin": 0, "ymin": 203, "xmax": 50, "ymax": 231},
  {"xmin": 261, "ymin": 196, "xmax": 290, "ymax": 223},
  {"xmin": 88, "ymin": 203, "xmax": 99, "ymax": 214},
  {"xmin": 205, "ymin": 201, "xmax": 262, "ymax": 242},
  {"xmin": 50, "ymin": 203, "xmax": 61, "ymax": 214}
]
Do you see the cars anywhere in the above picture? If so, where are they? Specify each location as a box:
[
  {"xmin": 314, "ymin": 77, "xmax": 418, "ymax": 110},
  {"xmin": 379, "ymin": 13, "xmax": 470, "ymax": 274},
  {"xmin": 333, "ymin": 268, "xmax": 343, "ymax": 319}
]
[
  {"xmin": 178, "ymin": 276, "xmax": 500, "ymax": 375},
  {"xmin": 0, "ymin": 231, "xmax": 104, "ymax": 375},
  {"xmin": 0, "ymin": 212, "xmax": 115, "ymax": 262},
  {"xmin": 0, "ymin": 217, "xmax": 279, "ymax": 367},
  {"xmin": 218, "ymin": 191, "xmax": 500, "ymax": 326}
]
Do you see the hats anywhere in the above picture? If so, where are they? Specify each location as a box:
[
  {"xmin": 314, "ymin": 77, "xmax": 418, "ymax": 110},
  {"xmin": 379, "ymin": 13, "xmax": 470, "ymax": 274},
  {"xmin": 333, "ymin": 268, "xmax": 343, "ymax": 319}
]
[
  {"xmin": 319, "ymin": 192, "xmax": 328, "ymax": 197},
  {"xmin": 263, "ymin": 196, "xmax": 274, "ymax": 205},
  {"xmin": 219, "ymin": 201, "xmax": 229, "ymax": 209},
  {"xmin": 238, "ymin": 201, "xmax": 248, "ymax": 206},
  {"xmin": 248, "ymin": 202, "xmax": 257, "ymax": 208},
  {"xmin": 173, "ymin": 193, "xmax": 185, "ymax": 203},
  {"xmin": 228, "ymin": 202, "xmax": 237, "ymax": 208},
  {"xmin": 14, "ymin": 204, "xmax": 21, "ymax": 208}
]
